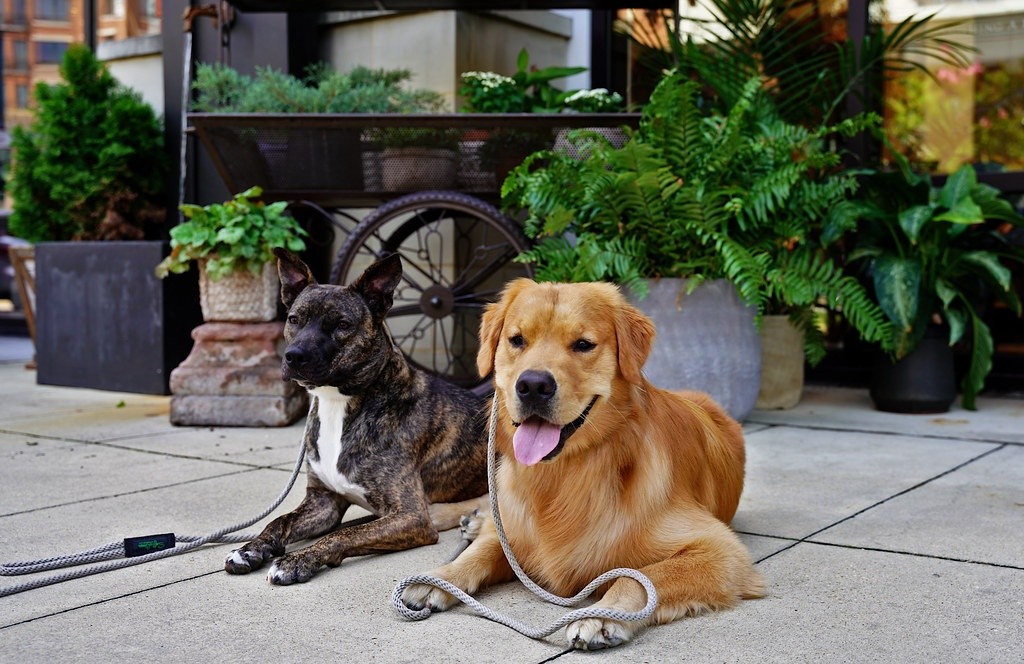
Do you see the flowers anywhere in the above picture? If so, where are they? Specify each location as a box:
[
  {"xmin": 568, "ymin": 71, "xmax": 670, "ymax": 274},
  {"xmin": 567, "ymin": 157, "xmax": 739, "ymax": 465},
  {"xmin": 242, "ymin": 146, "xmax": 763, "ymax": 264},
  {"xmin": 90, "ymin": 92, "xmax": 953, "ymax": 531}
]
[{"xmin": 368, "ymin": 57, "xmax": 625, "ymax": 152}]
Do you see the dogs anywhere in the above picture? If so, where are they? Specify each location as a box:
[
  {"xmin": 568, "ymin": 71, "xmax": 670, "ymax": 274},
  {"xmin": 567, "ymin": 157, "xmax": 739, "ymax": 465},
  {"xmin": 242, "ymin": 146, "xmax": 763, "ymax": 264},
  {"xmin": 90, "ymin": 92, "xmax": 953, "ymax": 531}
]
[
  {"xmin": 222, "ymin": 251, "xmax": 502, "ymax": 587},
  {"xmin": 402, "ymin": 278, "xmax": 769, "ymax": 650}
]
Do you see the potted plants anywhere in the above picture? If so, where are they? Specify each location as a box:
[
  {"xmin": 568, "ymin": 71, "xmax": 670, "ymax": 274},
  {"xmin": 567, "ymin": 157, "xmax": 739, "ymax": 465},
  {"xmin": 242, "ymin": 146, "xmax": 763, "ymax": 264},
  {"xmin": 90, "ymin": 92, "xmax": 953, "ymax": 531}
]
[
  {"xmin": 6, "ymin": 45, "xmax": 181, "ymax": 393},
  {"xmin": 525, "ymin": 0, "xmax": 1024, "ymax": 418},
  {"xmin": 156, "ymin": 180, "xmax": 301, "ymax": 321},
  {"xmin": 187, "ymin": 57, "xmax": 444, "ymax": 187}
]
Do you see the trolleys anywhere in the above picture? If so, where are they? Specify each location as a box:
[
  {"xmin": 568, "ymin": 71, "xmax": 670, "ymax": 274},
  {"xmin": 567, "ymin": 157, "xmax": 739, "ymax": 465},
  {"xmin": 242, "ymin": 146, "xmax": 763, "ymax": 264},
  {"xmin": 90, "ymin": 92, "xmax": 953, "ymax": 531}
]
[{"xmin": 176, "ymin": 110, "xmax": 646, "ymax": 387}]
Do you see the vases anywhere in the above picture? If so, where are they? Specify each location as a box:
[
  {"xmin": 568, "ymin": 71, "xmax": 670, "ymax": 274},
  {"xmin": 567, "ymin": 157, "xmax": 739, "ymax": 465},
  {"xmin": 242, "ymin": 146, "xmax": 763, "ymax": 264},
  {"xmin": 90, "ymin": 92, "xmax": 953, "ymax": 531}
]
[
  {"xmin": 378, "ymin": 143, "xmax": 459, "ymax": 190},
  {"xmin": 553, "ymin": 123, "xmax": 629, "ymax": 166},
  {"xmin": 461, "ymin": 139, "xmax": 489, "ymax": 170},
  {"xmin": 363, "ymin": 140, "xmax": 385, "ymax": 190},
  {"xmin": 462, "ymin": 123, "xmax": 505, "ymax": 171}
]
